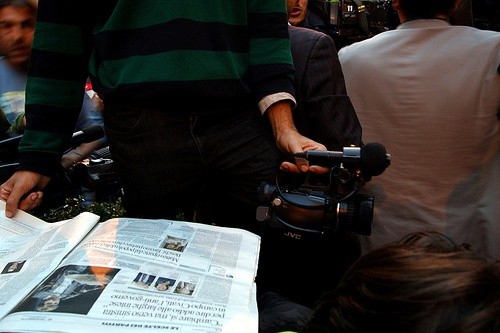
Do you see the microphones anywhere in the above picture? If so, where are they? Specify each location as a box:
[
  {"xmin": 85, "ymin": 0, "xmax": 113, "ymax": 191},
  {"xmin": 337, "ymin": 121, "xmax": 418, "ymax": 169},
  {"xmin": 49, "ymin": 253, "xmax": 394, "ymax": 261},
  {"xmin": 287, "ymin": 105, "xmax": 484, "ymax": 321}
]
[
  {"xmin": 293, "ymin": 144, "xmax": 392, "ymax": 176},
  {"xmin": 66, "ymin": 124, "xmax": 105, "ymax": 148}
]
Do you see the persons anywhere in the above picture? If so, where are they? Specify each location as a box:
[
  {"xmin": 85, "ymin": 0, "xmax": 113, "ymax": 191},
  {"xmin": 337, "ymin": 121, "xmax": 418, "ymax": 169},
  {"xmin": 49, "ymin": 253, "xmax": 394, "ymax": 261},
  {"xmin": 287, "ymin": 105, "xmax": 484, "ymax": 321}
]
[
  {"xmin": 0, "ymin": 0, "xmax": 500, "ymax": 333},
  {"xmin": 337, "ymin": 0, "xmax": 500, "ymax": 261}
]
